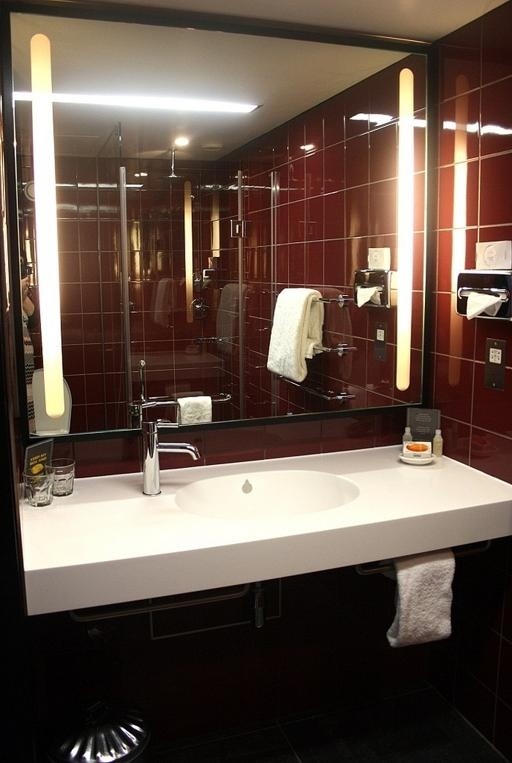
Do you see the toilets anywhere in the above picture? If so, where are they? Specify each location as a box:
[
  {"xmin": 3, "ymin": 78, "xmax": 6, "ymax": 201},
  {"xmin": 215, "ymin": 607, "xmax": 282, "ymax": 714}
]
[{"xmin": 32, "ymin": 368, "xmax": 73, "ymax": 436}]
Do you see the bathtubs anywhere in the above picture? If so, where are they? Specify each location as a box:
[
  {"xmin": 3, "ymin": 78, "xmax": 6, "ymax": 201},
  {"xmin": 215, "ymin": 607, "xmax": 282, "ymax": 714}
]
[{"xmin": 129, "ymin": 351, "xmax": 224, "ymax": 380}]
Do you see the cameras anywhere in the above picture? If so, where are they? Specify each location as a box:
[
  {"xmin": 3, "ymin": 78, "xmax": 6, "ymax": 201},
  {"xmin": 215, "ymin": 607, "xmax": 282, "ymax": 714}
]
[{"xmin": 21, "ymin": 261, "xmax": 32, "ymax": 279}]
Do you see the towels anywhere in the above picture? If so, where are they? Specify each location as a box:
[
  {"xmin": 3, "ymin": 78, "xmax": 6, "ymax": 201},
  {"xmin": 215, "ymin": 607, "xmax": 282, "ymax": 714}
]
[
  {"xmin": 386, "ymin": 547, "xmax": 457, "ymax": 649},
  {"xmin": 263, "ymin": 278, "xmax": 326, "ymax": 385},
  {"xmin": 175, "ymin": 393, "xmax": 212, "ymax": 426}
]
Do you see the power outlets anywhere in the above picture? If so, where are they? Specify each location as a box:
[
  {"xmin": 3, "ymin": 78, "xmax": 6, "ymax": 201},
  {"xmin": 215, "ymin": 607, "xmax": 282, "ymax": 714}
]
[{"xmin": 482, "ymin": 337, "xmax": 505, "ymax": 391}]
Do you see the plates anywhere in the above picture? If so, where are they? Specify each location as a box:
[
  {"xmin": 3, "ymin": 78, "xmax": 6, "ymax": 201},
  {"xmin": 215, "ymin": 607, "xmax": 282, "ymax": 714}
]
[{"xmin": 398, "ymin": 453, "xmax": 435, "ymax": 464}]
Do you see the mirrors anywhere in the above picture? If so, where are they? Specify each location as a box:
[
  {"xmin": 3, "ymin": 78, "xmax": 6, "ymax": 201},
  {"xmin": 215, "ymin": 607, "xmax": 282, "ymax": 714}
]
[{"xmin": 1, "ymin": 0, "xmax": 441, "ymax": 442}]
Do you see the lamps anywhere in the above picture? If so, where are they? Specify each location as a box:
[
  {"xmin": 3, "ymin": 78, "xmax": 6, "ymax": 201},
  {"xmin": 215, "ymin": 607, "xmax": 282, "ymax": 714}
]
[
  {"xmin": 27, "ymin": 25, "xmax": 67, "ymax": 419},
  {"xmin": 182, "ymin": 176, "xmax": 198, "ymax": 323},
  {"xmin": 393, "ymin": 63, "xmax": 415, "ymax": 392},
  {"xmin": 208, "ymin": 179, "xmax": 223, "ymax": 260}
]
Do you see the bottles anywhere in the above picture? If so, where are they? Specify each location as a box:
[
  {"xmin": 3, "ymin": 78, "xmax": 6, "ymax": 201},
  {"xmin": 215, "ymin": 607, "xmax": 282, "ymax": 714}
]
[
  {"xmin": 402, "ymin": 426, "xmax": 412, "ymax": 442},
  {"xmin": 432, "ymin": 429, "xmax": 443, "ymax": 456}
]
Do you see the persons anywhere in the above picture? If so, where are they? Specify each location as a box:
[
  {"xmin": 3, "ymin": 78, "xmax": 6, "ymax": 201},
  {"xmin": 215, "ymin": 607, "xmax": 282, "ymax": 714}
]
[{"xmin": 20, "ymin": 248, "xmax": 35, "ymax": 435}]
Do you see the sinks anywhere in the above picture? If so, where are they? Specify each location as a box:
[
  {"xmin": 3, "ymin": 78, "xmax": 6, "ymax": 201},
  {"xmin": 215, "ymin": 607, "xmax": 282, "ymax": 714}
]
[{"xmin": 172, "ymin": 469, "xmax": 363, "ymax": 525}]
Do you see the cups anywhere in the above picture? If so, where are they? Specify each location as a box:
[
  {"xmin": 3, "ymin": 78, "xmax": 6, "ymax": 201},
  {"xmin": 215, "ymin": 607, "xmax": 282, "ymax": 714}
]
[
  {"xmin": 21, "ymin": 467, "xmax": 55, "ymax": 507},
  {"xmin": 45, "ymin": 456, "xmax": 75, "ymax": 495}
]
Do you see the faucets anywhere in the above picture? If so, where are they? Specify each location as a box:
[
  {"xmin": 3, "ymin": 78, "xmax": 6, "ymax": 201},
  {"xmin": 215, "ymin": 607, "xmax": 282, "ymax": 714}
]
[{"xmin": 141, "ymin": 419, "xmax": 201, "ymax": 495}]
